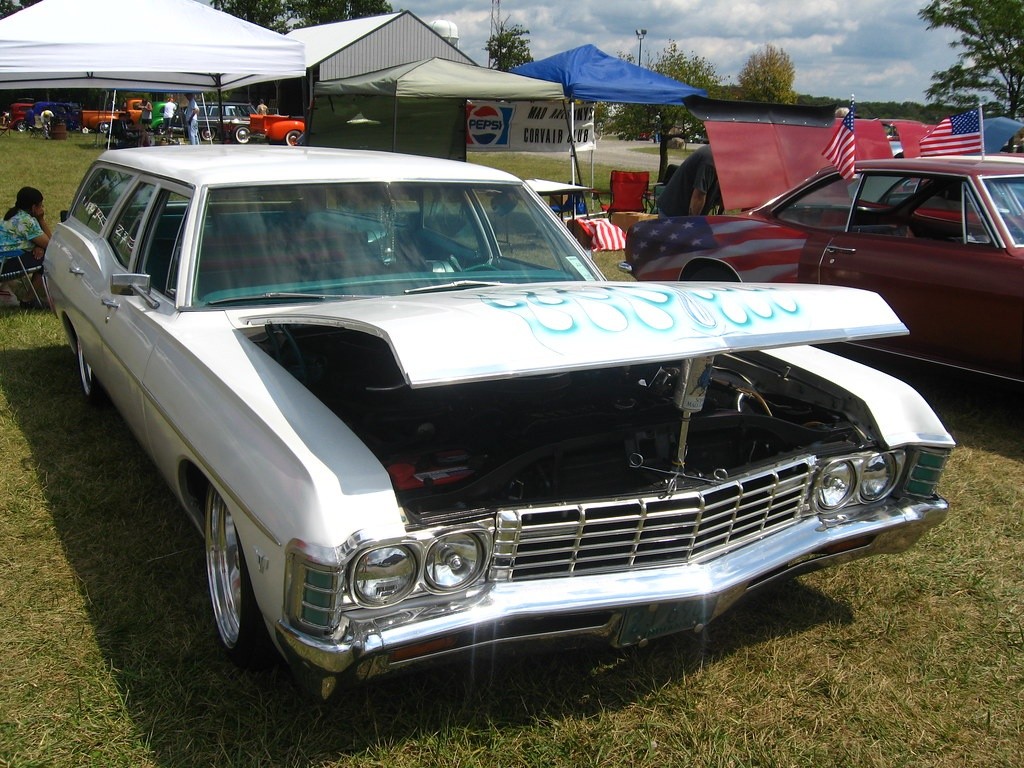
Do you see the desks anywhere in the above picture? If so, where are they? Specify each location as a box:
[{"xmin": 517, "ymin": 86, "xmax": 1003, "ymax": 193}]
[{"xmin": 524, "ymin": 178, "xmax": 595, "ymax": 222}]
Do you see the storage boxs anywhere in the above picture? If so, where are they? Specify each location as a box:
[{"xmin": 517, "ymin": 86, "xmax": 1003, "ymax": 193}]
[{"xmin": 549, "ymin": 194, "xmax": 659, "ymax": 251}]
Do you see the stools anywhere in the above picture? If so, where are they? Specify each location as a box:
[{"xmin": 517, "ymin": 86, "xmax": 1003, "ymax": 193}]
[
  {"xmin": 0, "ymin": 126, "xmax": 10, "ymax": 137},
  {"xmin": 29, "ymin": 125, "xmax": 45, "ymax": 138}
]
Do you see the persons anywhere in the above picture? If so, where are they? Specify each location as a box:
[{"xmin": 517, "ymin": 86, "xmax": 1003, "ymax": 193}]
[
  {"xmin": 656, "ymin": 143, "xmax": 723, "ymax": 221},
  {"xmin": 256, "ymin": 98, "xmax": 270, "ymax": 115},
  {"xmin": 40, "ymin": 109, "xmax": 53, "ymax": 140},
  {"xmin": 105, "ymin": 93, "xmax": 201, "ymax": 147},
  {"xmin": 0, "ymin": 186, "xmax": 52, "ymax": 308}
]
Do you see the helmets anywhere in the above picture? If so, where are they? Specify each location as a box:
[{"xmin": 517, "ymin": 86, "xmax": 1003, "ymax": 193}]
[{"xmin": 43, "ymin": 112, "xmax": 49, "ymax": 118}]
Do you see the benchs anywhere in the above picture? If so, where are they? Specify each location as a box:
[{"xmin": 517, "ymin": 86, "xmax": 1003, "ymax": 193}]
[
  {"xmin": 164, "ymin": 236, "xmax": 423, "ymax": 301},
  {"xmin": 112, "ymin": 211, "xmax": 309, "ymax": 244},
  {"xmin": 813, "ymin": 225, "xmax": 915, "ymax": 239}
]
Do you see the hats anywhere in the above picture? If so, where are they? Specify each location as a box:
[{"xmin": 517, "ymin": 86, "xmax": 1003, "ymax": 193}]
[{"xmin": 119, "ymin": 113, "xmax": 128, "ymax": 117}]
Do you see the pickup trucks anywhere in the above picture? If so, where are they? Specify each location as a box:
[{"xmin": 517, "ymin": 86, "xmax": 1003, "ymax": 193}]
[
  {"xmin": 248, "ymin": 113, "xmax": 305, "ymax": 146},
  {"xmin": 80, "ymin": 99, "xmax": 145, "ymax": 134}
]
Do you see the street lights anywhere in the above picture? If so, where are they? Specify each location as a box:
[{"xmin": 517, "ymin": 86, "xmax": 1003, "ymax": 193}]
[{"xmin": 634, "ymin": 29, "xmax": 647, "ymax": 67}]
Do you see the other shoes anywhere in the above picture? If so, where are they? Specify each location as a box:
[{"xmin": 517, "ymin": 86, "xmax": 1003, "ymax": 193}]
[
  {"xmin": 45, "ymin": 137, "xmax": 50, "ymax": 140},
  {"xmin": 20, "ymin": 297, "xmax": 50, "ymax": 310}
]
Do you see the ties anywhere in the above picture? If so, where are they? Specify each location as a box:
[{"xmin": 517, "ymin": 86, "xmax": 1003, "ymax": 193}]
[{"xmin": 123, "ymin": 121, "xmax": 126, "ymax": 130}]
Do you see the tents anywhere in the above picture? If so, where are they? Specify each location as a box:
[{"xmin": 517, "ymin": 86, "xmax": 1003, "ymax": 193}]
[
  {"xmin": 309, "ymin": 58, "xmax": 592, "ymax": 221},
  {"xmin": 511, "ymin": 45, "xmax": 704, "ymax": 221},
  {"xmin": 0, "ymin": 1, "xmax": 308, "ymax": 157}
]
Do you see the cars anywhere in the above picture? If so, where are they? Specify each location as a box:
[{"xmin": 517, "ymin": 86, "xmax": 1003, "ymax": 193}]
[
  {"xmin": 8, "ymin": 100, "xmax": 81, "ymax": 132},
  {"xmin": 618, "ymin": 94, "xmax": 1023, "ymax": 405},
  {"xmin": 139, "ymin": 100, "xmax": 258, "ymax": 142},
  {"xmin": 40, "ymin": 145, "xmax": 957, "ymax": 694}
]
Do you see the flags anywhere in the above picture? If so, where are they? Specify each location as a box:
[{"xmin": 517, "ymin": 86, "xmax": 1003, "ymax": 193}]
[
  {"xmin": 820, "ymin": 97, "xmax": 855, "ymax": 180},
  {"xmin": 918, "ymin": 113, "xmax": 984, "ymax": 159}
]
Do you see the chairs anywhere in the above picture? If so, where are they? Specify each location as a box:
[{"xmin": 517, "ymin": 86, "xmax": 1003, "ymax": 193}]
[
  {"xmin": 112, "ymin": 119, "xmax": 143, "ymax": 148},
  {"xmin": 589, "ymin": 169, "xmax": 649, "ymax": 224},
  {"xmin": 0, "ymin": 248, "xmax": 43, "ymax": 310}
]
